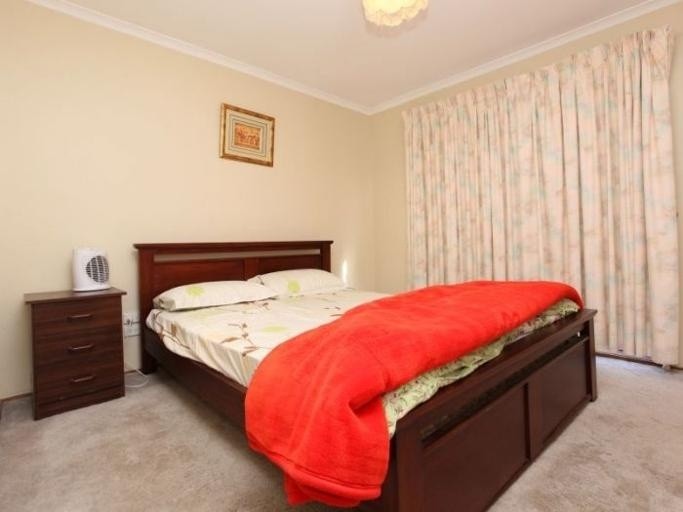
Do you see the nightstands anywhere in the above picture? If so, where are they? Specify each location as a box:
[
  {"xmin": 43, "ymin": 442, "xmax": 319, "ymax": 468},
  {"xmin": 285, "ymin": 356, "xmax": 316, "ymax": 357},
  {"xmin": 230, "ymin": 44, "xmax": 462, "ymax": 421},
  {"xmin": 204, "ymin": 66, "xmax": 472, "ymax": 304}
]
[{"xmin": 24, "ymin": 287, "xmax": 128, "ymax": 422}]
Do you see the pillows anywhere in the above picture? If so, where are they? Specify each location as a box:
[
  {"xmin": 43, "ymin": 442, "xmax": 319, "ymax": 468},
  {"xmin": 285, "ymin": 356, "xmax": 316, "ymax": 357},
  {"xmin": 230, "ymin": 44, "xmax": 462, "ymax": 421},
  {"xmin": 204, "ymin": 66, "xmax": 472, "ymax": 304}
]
[{"xmin": 152, "ymin": 267, "xmax": 347, "ymax": 313}]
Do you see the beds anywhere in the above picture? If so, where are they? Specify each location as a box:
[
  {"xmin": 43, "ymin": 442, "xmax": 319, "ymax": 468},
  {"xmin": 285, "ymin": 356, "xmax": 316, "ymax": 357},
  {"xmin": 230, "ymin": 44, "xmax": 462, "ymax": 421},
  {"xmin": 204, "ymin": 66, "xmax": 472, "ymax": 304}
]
[{"xmin": 131, "ymin": 239, "xmax": 599, "ymax": 512}]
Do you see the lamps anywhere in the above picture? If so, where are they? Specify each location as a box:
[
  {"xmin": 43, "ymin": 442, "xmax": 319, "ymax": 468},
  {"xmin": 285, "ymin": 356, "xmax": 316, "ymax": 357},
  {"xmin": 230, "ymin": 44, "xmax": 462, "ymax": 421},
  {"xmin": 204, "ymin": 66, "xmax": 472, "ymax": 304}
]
[{"xmin": 362, "ymin": 1, "xmax": 429, "ymax": 29}]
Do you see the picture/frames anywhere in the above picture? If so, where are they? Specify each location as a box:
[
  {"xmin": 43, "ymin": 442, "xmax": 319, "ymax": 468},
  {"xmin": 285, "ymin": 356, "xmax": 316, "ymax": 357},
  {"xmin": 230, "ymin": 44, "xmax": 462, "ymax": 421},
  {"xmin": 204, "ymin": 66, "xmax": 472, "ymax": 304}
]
[{"xmin": 219, "ymin": 103, "xmax": 276, "ymax": 167}]
